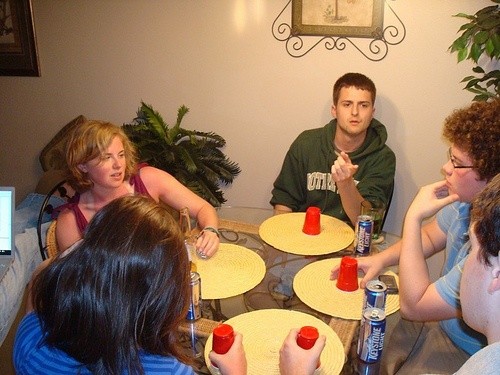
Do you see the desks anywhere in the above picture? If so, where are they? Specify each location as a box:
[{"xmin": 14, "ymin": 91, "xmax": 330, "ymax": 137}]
[{"xmin": 173, "ymin": 207, "xmax": 426, "ymax": 375}]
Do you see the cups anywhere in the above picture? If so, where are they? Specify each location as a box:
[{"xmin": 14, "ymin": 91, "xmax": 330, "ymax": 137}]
[
  {"xmin": 303, "ymin": 205, "xmax": 321, "ymax": 235},
  {"xmin": 210, "ymin": 324, "xmax": 234, "ymax": 369},
  {"xmin": 337, "ymin": 255, "xmax": 359, "ymax": 292},
  {"xmin": 297, "ymin": 326, "xmax": 321, "ymax": 369},
  {"xmin": 360, "ymin": 198, "xmax": 387, "ymax": 240}
]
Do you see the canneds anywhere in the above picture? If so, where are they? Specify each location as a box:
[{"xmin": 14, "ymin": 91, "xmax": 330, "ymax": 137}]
[
  {"xmin": 185, "ymin": 272, "xmax": 202, "ymax": 321},
  {"xmin": 188, "ymin": 324, "xmax": 202, "ymax": 358},
  {"xmin": 356, "ymin": 307, "xmax": 386, "ymax": 363},
  {"xmin": 363, "ymin": 279, "xmax": 387, "ymax": 310},
  {"xmin": 358, "ymin": 362, "xmax": 380, "ymax": 375},
  {"xmin": 354, "ymin": 215, "xmax": 373, "ymax": 255}
]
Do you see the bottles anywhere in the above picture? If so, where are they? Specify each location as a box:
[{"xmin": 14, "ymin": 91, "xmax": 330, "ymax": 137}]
[{"xmin": 179, "ymin": 208, "xmax": 197, "ymax": 271}]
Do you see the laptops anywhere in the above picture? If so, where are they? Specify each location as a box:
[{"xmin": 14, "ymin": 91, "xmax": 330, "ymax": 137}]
[{"xmin": 0, "ymin": 186, "xmax": 15, "ymax": 282}]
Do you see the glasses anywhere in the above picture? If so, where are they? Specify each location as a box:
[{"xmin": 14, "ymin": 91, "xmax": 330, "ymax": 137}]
[{"xmin": 448, "ymin": 147, "xmax": 480, "ymax": 169}]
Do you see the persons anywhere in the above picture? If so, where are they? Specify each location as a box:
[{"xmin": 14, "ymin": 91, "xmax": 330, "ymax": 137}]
[
  {"xmin": 330, "ymin": 101, "xmax": 500, "ymax": 356},
  {"xmin": 269, "ymin": 72, "xmax": 396, "ymax": 232},
  {"xmin": 56, "ymin": 120, "xmax": 220, "ymax": 258},
  {"xmin": 11, "ymin": 194, "xmax": 248, "ymax": 375},
  {"xmin": 279, "ymin": 172, "xmax": 500, "ymax": 375}
]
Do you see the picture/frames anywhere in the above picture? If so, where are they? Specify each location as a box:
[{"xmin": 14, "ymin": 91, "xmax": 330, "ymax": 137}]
[
  {"xmin": 291, "ymin": 0, "xmax": 385, "ymax": 39},
  {"xmin": 0, "ymin": 0, "xmax": 42, "ymax": 77}
]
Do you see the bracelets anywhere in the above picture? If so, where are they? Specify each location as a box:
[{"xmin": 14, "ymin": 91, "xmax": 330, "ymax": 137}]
[{"xmin": 196, "ymin": 225, "xmax": 220, "ymax": 239}]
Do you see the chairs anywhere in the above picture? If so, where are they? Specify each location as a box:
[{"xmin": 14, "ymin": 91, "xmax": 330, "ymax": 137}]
[{"xmin": 37, "ymin": 180, "xmax": 80, "ymax": 261}]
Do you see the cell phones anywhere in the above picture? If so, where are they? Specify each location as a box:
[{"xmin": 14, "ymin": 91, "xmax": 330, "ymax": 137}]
[{"xmin": 378, "ymin": 274, "xmax": 399, "ymax": 294}]
